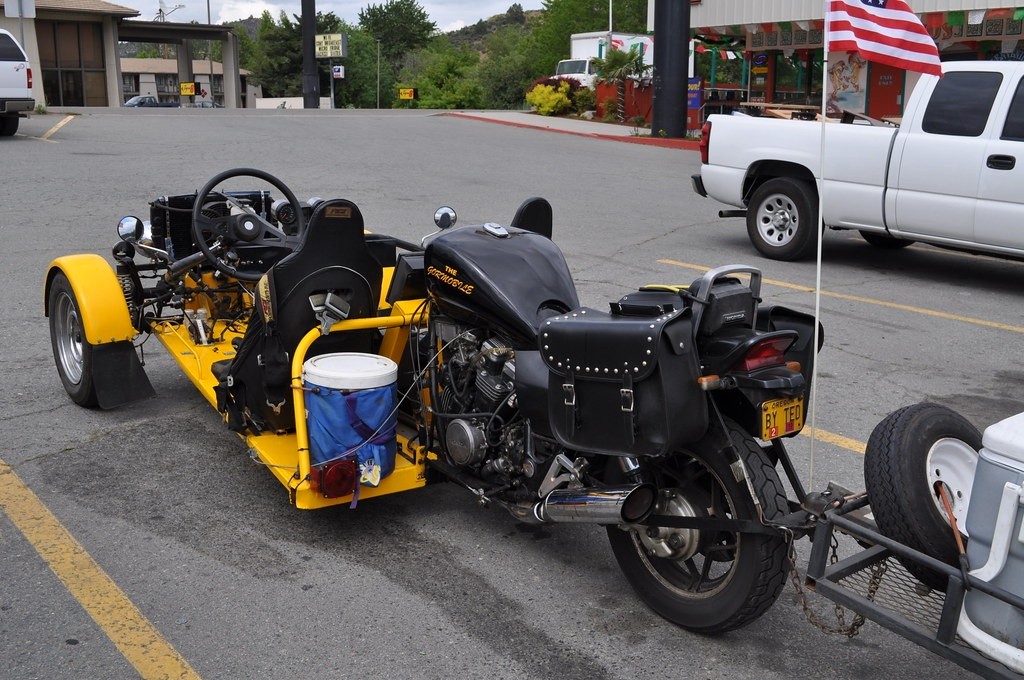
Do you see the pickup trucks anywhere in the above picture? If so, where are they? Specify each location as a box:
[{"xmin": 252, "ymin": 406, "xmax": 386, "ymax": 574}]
[
  {"xmin": 691, "ymin": 61, "xmax": 1024, "ymax": 262},
  {"xmin": 122, "ymin": 94, "xmax": 180, "ymax": 108}
]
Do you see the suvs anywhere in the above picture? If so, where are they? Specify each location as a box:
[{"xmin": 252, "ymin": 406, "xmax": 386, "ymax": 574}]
[{"xmin": 0, "ymin": 29, "xmax": 35, "ymax": 138}]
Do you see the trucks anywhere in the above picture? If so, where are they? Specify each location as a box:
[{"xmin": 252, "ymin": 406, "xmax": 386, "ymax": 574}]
[{"xmin": 550, "ymin": 30, "xmax": 654, "ymax": 92}]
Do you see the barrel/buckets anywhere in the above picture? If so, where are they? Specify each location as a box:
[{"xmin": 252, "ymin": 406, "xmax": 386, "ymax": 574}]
[{"xmin": 300, "ymin": 351, "xmax": 397, "ymax": 486}]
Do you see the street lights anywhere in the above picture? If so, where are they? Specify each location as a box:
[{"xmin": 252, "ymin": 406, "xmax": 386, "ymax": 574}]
[{"xmin": 376, "ymin": 39, "xmax": 381, "ymax": 109}]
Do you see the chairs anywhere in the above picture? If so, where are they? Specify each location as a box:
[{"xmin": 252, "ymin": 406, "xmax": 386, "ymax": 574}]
[
  {"xmin": 211, "ymin": 200, "xmax": 382, "ymax": 437},
  {"xmin": 509, "ymin": 196, "xmax": 554, "ymax": 242}
]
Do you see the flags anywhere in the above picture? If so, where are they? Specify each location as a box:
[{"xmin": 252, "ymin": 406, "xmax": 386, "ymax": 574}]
[
  {"xmin": 690, "ymin": 7, "xmax": 1024, "ymax": 65},
  {"xmin": 825, "ymin": 0, "xmax": 943, "ymax": 77}
]
